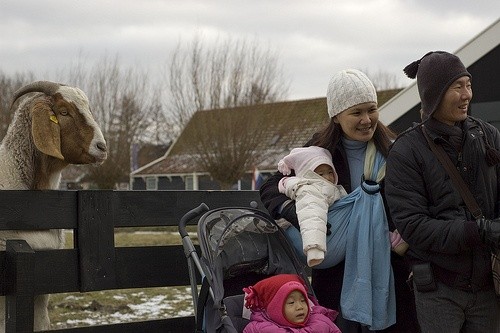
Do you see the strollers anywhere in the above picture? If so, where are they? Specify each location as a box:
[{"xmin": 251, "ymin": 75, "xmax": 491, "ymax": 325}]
[{"xmin": 178, "ymin": 201, "xmax": 318, "ymax": 333}]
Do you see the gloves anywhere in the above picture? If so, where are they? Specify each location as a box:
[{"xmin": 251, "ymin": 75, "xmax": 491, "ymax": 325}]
[{"xmin": 391, "ymin": 229, "xmax": 410, "ymax": 257}]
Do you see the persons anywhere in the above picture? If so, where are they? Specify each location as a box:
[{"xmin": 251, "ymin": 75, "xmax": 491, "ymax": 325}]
[
  {"xmin": 277, "ymin": 145, "xmax": 348, "ymax": 267},
  {"xmin": 261, "ymin": 68, "xmax": 409, "ymax": 333},
  {"xmin": 383, "ymin": 52, "xmax": 500, "ymax": 333},
  {"xmin": 243, "ymin": 273, "xmax": 342, "ymax": 332}
]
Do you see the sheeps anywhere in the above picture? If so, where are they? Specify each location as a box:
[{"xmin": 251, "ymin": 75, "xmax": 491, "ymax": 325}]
[{"xmin": 0, "ymin": 79, "xmax": 110, "ymax": 333}]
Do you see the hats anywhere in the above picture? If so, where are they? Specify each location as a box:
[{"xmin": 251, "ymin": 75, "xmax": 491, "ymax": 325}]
[
  {"xmin": 326, "ymin": 69, "xmax": 377, "ymax": 119},
  {"xmin": 387, "ymin": 51, "xmax": 472, "ymax": 154},
  {"xmin": 277, "ymin": 145, "xmax": 338, "ymax": 185}
]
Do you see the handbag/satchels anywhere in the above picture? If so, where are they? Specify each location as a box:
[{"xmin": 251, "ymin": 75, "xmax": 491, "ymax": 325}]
[
  {"xmin": 413, "ymin": 261, "xmax": 437, "ymax": 293},
  {"xmin": 491, "ymin": 252, "xmax": 500, "ymax": 299}
]
